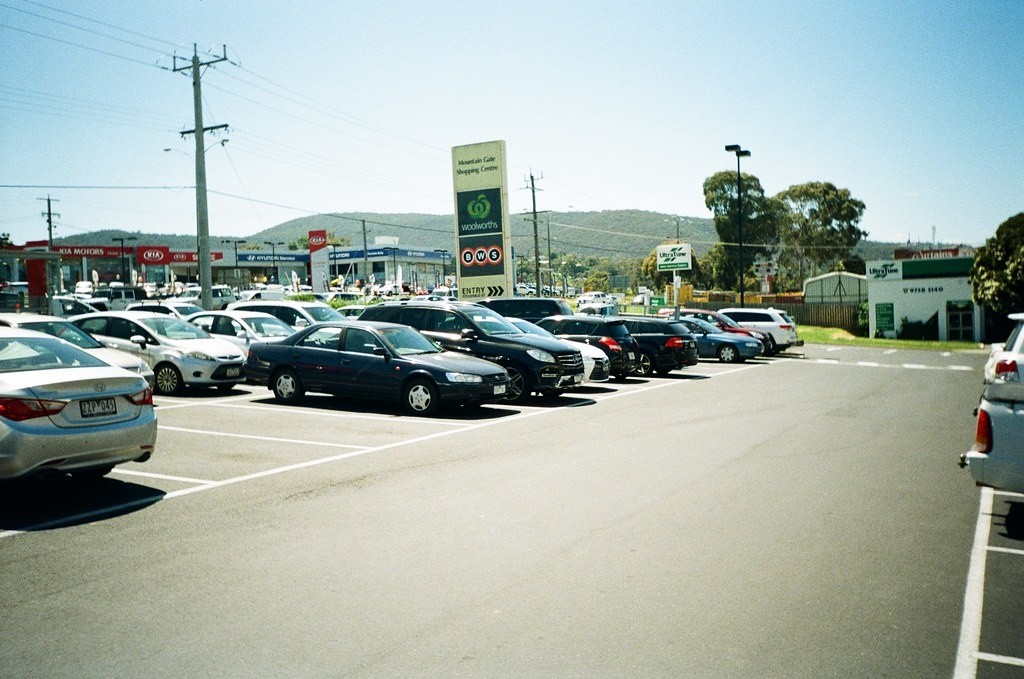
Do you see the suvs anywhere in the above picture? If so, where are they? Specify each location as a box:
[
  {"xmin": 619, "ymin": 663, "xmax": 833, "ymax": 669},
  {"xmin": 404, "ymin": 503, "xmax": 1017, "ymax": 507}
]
[{"xmin": 957, "ymin": 312, "xmax": 1024, "ymax": 497}]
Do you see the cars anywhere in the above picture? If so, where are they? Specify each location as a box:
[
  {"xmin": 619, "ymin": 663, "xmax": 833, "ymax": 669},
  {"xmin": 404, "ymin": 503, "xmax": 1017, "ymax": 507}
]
[
  {"xmin": 356, "ymin": 300, "xmax": 585, "ymax": 402},
  {"xmin": 67, "ymin": 310, "xmax": 248, "ymax": 395},
  {"xmin": 0, "ymin": 313, "xmax": 155, "ymax": 393},
  {"xmin": 247, "ymin": 320, "xmax": 510, "ymax": 416},
  {"xmin": 183, "ymin": 310, "xmax": 307, "ymax": 364},
  {"xmin": 0, "ymin": 326, "xmax": 158, "ymax": 485},
  {"xmin": 667, "ymin": 307, "xmax": 797, "ymax": 363},
  {"xmin": 46, "ymin": 281, "xmax": 620, "ymax": 321},
  {"xmin": 501, "ymin": 316, "xmax": 640, "ymax": 384},
  {"xmin": 619, "ymin": 317, "xmax": 699, "ymax": 377}
]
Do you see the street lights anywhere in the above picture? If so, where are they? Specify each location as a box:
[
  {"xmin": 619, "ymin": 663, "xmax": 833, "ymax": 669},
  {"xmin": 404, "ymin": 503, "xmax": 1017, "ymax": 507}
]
[
  {"xmin": 724, "ymin": 143, "xmax": 752, "ymax": 306},
  {"xmin": 221, "ymin": 240, "xmax": 246, "ymax": 287},
  {"xmin": 434, "ymin": 249, "xmax": 448, "ymax": 287},
  {"xmin": 113, "ymin": 237, "xmax": 138, "ymax": 287},
  {"xmin": 326, "ymin": 244, "xmax": 342, "ymax": 279},
  {"xmin": 383, "ymin": 247, "xmax": 398, "ymax": 299},
  {"xmin": 264, "ymin": 242, "xmax": 285, "ymax": 285}
]
